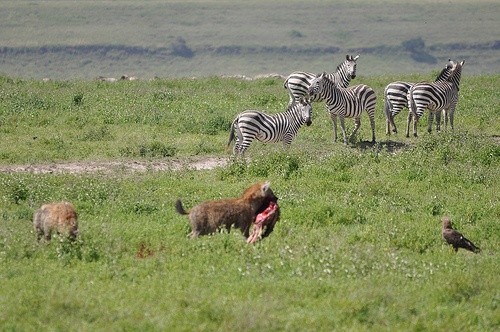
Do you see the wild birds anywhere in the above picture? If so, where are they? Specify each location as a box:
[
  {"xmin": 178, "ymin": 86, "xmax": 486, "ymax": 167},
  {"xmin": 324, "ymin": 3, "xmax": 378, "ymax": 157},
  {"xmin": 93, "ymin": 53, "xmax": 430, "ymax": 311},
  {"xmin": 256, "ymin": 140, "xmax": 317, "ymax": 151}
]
[{"xmin": 441, "ymin": 217, "xmax": 481, "ymax": 255}]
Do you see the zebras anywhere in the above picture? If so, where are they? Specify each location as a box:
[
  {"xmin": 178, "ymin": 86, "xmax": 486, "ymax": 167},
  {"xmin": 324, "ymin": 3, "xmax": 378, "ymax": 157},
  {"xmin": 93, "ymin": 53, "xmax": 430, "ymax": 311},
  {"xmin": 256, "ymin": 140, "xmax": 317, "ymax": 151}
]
[
  {"xmin": 383, "ymin": 58, "xmax": 465, "ymax": 138},
  {"xmin": 283, "ymin": 54, "xmax": 377, "ymax": 145},
  {"xmin": 226, "ymin": 97, "xmax": 313, "ymax": 157}
]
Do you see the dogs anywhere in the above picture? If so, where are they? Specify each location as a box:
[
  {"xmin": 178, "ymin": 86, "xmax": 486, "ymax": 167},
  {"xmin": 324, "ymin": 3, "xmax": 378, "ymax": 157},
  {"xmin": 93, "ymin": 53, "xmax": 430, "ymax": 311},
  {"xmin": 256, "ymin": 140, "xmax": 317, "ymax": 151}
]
[
  {"xmin": 174, "ymin": 181, "xmax": 278, "ymax": 240},
  {"xmin": 30, "ymin": 201, "xmax": 79, "ymax": 243}
]
